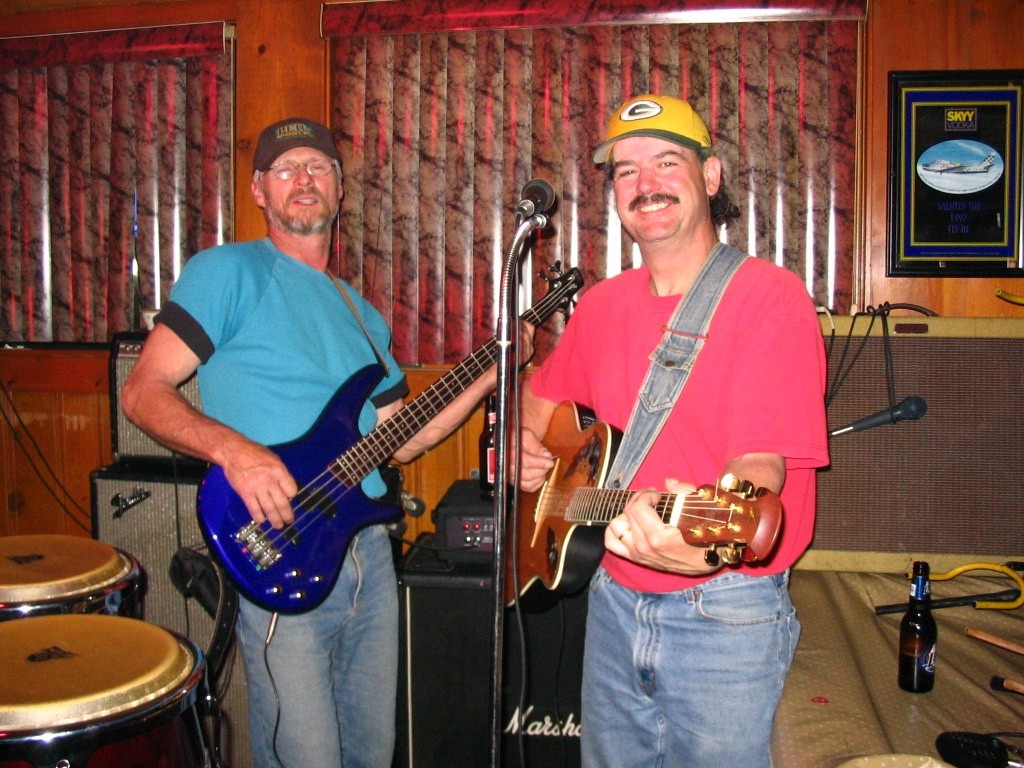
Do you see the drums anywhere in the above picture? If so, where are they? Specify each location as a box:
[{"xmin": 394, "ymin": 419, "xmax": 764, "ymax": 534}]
[
  {"xmin": 0, "ymin": 534, "xmax": 141, "ymax": 625},
  {"xmin": 0, "ymin": 613, "xmax": 209, "ymax": 768}
]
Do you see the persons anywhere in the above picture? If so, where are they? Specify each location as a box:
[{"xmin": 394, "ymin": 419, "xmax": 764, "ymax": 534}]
[
  {"xmin": 122, "ymin": 118, "xmax": 536, "ymax": 768},
  {"xmin": 509, "ymin": 93, "xmax": 828, "ymax": 767}
]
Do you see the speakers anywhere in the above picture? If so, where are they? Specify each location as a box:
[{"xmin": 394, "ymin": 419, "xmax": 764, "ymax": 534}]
[
  {"xmin": 108, "ymin": 331, "xmax": 200, "ymax": 475},
  {"xmin": 397, "ymin": 528, "xmax": 588, "ymax": 768},
  {"xmin": 87, "ymin": 469, "xmax": 250, "ymax": 768}
]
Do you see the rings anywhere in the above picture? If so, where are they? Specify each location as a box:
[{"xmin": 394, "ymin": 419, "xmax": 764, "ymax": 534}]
[{"xmin": 618, "ymin": 528, "xmax": 630, "ymax": 540}]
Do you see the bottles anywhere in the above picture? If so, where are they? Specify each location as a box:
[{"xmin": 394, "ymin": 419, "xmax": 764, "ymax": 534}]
[
  {"xmin": 897, "ymin": 561, "xmax": 937, "ymax": 694},
  {"xmin": 479, "ymin": 396, "xmax": 497, "ymax": 503}
]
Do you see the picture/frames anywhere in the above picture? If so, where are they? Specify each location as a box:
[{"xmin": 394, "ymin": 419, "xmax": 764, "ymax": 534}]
[{"xmin": 887, "ymin": 68, "xmax": 1024, "ymax": 278}]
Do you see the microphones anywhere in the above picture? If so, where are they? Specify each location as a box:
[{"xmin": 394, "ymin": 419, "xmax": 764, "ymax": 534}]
[
  {"xmin": 513, "ymin": 176, "xmax": 557, "ymax": 219},
  {"xmin": 828, "ymin": 394, "xmax": 928, "ymax": 438}
]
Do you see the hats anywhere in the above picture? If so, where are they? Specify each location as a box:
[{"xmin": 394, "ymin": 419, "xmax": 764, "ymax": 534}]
[
  {"xmin": 252, "ymin": 116, "xmax": 343, "ymax": 175},
  {"xmin": 591, "ymin": 95, "xmax": 712, "ymax": 164}
]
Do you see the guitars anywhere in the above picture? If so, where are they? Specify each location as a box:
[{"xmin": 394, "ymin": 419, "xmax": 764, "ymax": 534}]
[
  {"xmin": 503, "ymin": 399, "xmax": 784, "ymax": 609},
  {"xmin": 195, "ymin": 259, "xmax": 586, "ymax": 612}
]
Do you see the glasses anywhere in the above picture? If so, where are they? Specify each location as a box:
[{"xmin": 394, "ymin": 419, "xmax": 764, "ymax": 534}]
[{"xmin": 258, "ymin": 158, "xmax": 336, "ymax": 181}]
